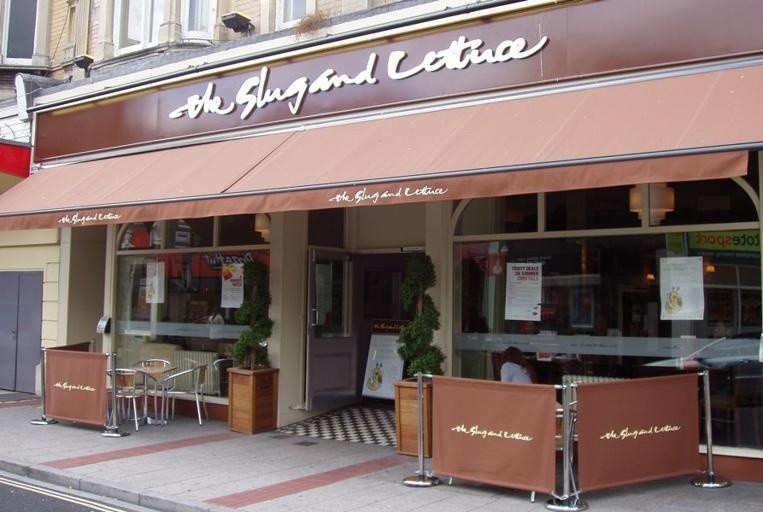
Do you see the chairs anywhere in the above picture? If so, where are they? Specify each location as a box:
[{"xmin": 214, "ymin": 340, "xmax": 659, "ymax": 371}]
[
  {"xmin": 106, "ymin": 356, "xmax": 209, "ymax": 431},
  {"xmin": 556, "ymin": 381, "xmax": 579, "ymax": 502}
]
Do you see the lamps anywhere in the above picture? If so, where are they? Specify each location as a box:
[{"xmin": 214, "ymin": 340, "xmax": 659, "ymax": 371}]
[
  {"xmin": 74, "ymin": 55, "xmax": 94, "ymax": 71},
  {"xmin": 629, "ymin": 182, "xmax": 675, "ymax": 226},
  {"xmin": 220, "ymin": 13, "xmax": 255, "ymax": 33},
  {"xmin": 254, "ymin": 213, "xmax": 271, "ymax": 243}
]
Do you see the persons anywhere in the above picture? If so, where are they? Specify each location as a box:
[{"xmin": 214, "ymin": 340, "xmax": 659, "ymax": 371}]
[
  {"xmin": 206, "ymin": 308, "xmax": 224, "ymax": 324},
  {"xmin": 499, "ymin": 345, "xmax": 538, "ymax": 385}
]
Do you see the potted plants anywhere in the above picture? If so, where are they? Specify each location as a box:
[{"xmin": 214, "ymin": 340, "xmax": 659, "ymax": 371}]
[
  {"xmin": 393, "ymin": 252, "xmax": 448, "ymax": 458},
  {"xmin": 226, "ymin": 260, "xmax": 279, "ymax": 435}
]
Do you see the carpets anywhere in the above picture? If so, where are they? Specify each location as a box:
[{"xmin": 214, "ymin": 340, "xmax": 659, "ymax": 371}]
[{"xmin": 277, "ymin": 404, "xmax": 396, "ymax": 448}]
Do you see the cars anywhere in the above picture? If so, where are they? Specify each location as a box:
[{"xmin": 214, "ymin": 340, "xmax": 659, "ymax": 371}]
[{"xmin": 640, "ymin": 329, "xmax": 763, "ymax": 408}]
